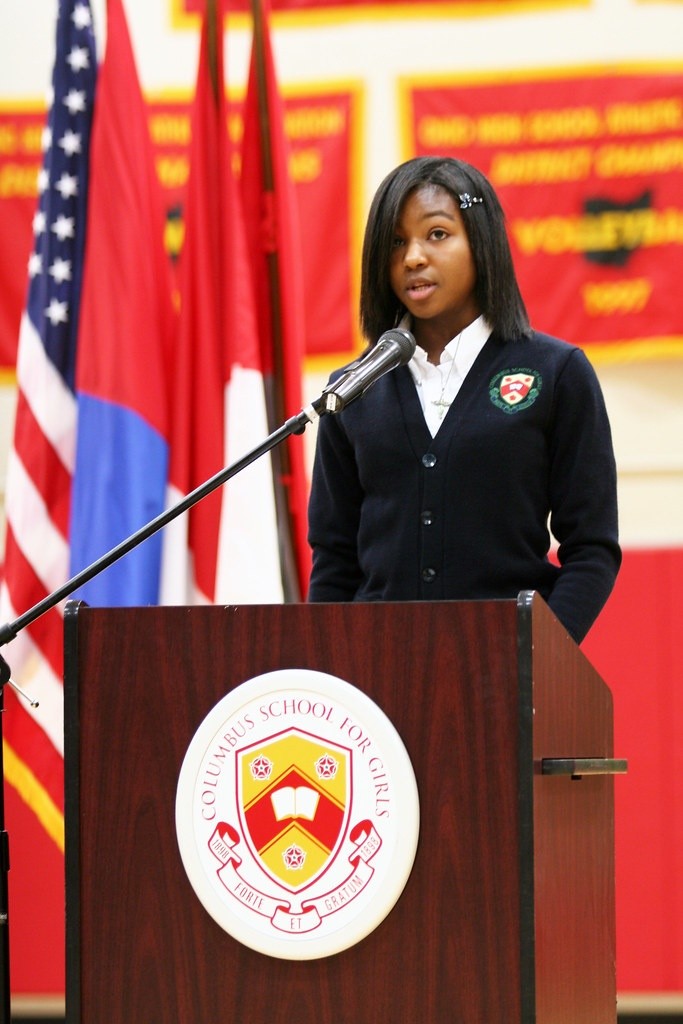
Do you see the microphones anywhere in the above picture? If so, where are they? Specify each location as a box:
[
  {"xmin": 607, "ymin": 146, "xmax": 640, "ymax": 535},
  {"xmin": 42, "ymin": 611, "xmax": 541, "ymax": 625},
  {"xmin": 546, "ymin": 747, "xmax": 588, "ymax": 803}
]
[{"xmin": 320, "ymin": 329, "xmax": 417, "ymax": 416}]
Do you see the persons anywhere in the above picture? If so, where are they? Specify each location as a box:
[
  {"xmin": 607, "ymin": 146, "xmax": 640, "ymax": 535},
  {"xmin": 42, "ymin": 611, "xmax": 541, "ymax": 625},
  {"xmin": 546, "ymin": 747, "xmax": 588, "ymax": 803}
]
[{"xmin": 304, "ymin": 160, "xmax": 622, "ymax": 647}]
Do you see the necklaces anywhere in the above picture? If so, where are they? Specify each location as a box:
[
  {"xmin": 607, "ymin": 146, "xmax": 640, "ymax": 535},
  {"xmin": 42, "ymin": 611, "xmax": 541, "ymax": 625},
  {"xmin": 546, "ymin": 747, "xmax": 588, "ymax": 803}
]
[{"xmin": 429, "ymin": 330, "xmax": 463, "ymax": 420}]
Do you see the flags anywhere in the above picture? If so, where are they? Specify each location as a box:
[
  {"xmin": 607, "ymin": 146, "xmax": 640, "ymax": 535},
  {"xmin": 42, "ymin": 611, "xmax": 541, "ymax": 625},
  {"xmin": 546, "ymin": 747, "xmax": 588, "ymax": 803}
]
[{"xmin": 0, "ymin": 0, "xmax": 310, "ymax": 1000}]
